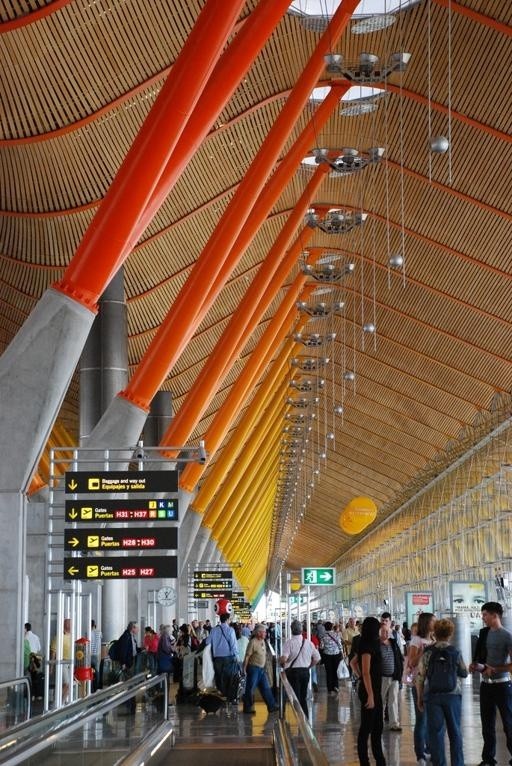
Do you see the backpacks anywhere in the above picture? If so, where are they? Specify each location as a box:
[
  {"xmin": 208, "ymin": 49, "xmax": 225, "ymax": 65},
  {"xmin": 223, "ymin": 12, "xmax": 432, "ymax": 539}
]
[
  {"xmin": 107, "ymin": 638, "xmax": 121, "ymax": 662},
  {"xmin": 425, "ymin": 645, "xmax": 462, "ymax": 695}
]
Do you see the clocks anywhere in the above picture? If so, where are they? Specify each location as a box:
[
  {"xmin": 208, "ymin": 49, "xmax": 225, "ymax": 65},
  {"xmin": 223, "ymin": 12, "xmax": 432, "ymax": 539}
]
[{"xmin": 156, "ymin": 586, "xmax": 178, "ymax": 607}]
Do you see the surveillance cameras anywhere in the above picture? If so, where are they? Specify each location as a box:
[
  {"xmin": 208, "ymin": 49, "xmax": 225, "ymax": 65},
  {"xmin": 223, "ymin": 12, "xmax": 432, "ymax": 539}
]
[
  {"xmin": 198, "ymin": 446, "xmax": 206, "ymax": 462},
  {"xmin": 135, "ymin": 448, "xmax": 144, "ymax": 459}
]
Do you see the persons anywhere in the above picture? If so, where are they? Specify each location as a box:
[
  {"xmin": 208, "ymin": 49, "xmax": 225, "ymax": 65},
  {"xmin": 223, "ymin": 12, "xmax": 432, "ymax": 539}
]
[
  {"xmin": 405, "ymin": 612, "xmax": 440, "ymax": 766},
  {"xmin": 379, "ymin": 734, "xmax": 402, "ymax": 766},
  {"xmin": 415, "ymin": 617, "xmax": 469, "ymax": 766},
  {"xmin": 320, "ymin": 693, "xmax": 346, "ymax": 766},
  {"xmin": 116, "ymin": 611, "xmax": 423, "ymax": 731},
  {"xmin": 350, "ymin": 615, "xmax": 390, "ymax": 766},
  {"xmin": 48, "ymin": 619, "xmax": 71, "ymax": 704},
  {"xmin": 90, "ymin": 619, "xmax": 101, "ymax": 694},
  {"xmin": 24, "ymin": 623, "xmax": 43, "ymax": 702},
  {"xmin": 453, "ymin": 581, "xmax": 485, "ymax": 636},
  {"xmin": 468, "ymin": 600, "xmax": 511, "ymax": 766}
]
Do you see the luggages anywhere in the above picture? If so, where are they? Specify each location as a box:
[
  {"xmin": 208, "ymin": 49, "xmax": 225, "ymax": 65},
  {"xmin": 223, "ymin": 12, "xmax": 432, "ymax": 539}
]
[{"xmin": 108, "ymin": 668, "xmax": 125, "ymax": 685}]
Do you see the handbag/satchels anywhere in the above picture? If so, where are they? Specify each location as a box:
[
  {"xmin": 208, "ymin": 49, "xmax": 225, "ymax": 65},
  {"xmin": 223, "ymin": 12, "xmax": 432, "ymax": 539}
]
[{"xmin": 230, "ymin": 661, "xmax": 247, "ymax": 700}]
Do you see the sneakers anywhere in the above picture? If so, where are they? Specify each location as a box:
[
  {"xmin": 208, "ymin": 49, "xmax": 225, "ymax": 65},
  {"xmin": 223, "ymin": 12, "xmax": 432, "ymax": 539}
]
[{"xmin": 245, "ymin": 705, "xmax": 280, "ymax": 717}]
